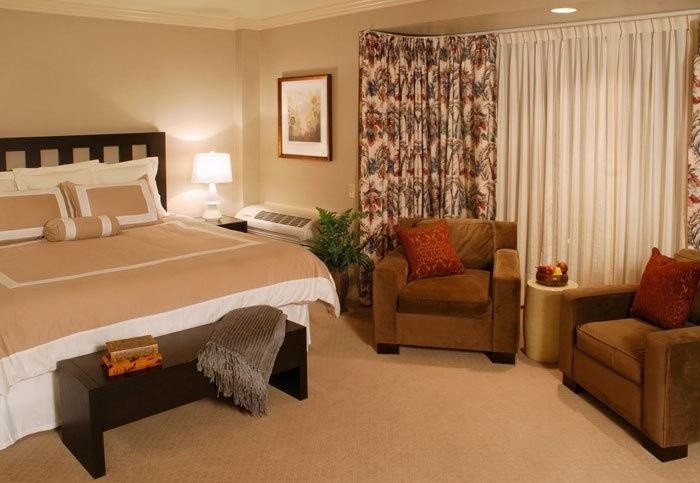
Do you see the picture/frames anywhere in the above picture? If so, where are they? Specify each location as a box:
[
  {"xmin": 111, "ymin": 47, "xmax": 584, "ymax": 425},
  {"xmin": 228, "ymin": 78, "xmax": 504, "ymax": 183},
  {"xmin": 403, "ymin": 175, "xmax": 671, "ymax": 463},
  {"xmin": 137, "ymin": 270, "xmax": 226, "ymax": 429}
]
[{"xmin": 277, "ymin": 71, "xmax": 332, "ymax": 162}]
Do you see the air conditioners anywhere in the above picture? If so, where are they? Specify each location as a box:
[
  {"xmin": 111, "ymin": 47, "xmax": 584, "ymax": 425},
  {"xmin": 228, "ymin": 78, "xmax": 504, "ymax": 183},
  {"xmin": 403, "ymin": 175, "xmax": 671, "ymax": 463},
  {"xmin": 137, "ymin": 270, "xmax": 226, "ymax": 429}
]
[{"xmin": 234, "ymin": 203, "xmax": 323, "ymax": 247}]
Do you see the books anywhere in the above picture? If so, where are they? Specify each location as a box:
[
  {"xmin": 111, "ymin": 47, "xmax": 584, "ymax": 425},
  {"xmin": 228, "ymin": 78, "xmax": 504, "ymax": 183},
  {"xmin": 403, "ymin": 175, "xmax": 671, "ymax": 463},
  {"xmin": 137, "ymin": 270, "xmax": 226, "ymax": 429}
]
[
  {"xmin": 100, "ymin": 352, "xmax": 163, "ymax": 377},
  {"xmin": 106, "ymin": 334, "xmax": 159, "ymax": 361}
]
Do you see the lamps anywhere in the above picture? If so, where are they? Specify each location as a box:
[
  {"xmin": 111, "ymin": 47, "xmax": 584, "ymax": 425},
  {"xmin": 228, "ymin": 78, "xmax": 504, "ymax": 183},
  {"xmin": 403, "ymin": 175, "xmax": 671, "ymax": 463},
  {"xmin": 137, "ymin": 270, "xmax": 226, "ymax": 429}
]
[{"xmin": 189, "ymin": 149, "xmax": 235, "ymax": 222}]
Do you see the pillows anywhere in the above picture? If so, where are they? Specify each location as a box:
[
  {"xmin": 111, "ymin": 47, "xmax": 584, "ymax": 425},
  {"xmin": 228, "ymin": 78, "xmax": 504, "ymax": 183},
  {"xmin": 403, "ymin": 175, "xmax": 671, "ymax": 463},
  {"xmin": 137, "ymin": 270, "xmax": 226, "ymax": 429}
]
[
  {"xmin": 390, "ymin": 216, "xmax": 467, "ymax": 283},
  {"xmin": 0, "ymin": 155, "xmax": 172, "ymax": 241},
  {"xmin": 625, "ymin": 244, "xmax": 700, "ymax": 330}
]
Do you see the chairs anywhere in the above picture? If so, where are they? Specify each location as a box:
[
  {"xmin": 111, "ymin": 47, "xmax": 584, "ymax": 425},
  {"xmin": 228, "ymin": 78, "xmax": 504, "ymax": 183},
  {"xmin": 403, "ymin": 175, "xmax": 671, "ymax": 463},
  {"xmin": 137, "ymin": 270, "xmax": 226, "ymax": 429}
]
[
  {"xmin": 556, "ymin": 246, "xmax": 700, "ymax": 463},
  {"xmin": 368, "ymin": 216, "xmax": 523, "ymax": 367}
]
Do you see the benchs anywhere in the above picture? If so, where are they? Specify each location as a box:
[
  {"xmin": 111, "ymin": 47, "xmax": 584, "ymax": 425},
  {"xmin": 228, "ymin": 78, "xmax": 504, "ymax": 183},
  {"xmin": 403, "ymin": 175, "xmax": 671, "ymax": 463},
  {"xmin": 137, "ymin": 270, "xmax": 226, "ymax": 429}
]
[{"xmin": 52, "ymin": 312, "xmax": 312, "ymax": 479}]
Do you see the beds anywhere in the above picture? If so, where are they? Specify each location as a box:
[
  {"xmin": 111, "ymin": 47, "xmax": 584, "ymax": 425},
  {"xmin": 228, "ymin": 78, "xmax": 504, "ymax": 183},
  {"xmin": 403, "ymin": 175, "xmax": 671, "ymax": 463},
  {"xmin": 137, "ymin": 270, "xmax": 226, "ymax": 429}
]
[{"xmin": 0, "ymin": 132, "xmax": 343, "ymax": 454}]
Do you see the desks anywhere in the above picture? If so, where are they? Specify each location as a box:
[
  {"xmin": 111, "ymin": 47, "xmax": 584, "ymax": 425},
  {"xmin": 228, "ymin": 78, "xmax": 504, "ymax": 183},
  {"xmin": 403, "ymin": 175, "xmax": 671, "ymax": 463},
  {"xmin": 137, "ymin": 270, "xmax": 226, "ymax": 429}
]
[{"xmin": 522, "ymin": 275, "xmax": 579, "ymax": 364}]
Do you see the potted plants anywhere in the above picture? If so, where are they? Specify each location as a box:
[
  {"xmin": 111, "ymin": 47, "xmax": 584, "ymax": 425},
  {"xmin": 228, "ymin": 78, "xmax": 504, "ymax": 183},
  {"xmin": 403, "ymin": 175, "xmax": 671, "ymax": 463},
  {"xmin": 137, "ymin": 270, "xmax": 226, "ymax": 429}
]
[{"xmin": 299, "ymin": 203, "xmax": 376, "ymax": 315}]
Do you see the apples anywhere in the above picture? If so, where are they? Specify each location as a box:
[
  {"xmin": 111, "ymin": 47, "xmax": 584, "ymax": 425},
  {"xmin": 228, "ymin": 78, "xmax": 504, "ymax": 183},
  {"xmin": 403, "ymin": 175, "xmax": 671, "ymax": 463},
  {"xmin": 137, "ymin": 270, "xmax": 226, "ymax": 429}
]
[{"xmin": 558, "ymin": 262, "xmax": 568, "ymax": 274}]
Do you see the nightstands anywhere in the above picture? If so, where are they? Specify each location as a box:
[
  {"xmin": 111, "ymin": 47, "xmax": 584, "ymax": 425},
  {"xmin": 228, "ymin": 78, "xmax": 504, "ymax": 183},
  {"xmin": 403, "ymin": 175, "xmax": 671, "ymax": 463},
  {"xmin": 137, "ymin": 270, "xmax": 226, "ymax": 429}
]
[{"xmin": 193, "ymin": 213, "xmax": 249, "ymax": 234}]
